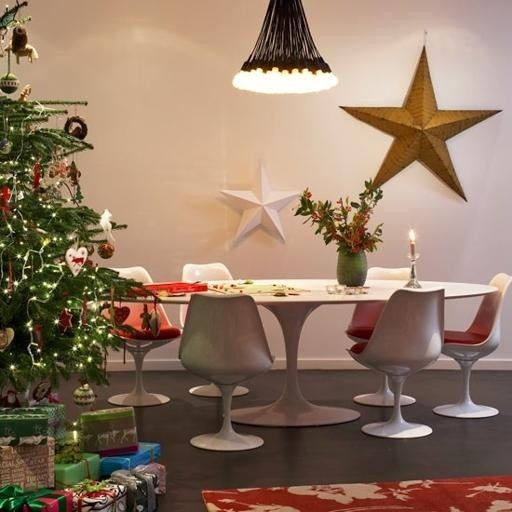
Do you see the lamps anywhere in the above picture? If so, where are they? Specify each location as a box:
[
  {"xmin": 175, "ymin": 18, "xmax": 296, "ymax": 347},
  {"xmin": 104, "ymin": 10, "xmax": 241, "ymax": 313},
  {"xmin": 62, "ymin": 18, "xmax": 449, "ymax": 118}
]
[{"xmin": 232, "ymin": 0, "xmax": 339, "ymax": 95}]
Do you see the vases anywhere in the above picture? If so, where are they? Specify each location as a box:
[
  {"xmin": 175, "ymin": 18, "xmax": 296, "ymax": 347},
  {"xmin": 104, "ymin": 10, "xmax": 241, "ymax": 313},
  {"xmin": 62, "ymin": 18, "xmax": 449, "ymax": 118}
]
[{"xmin": 336, "ymin": 248, "xmax": 368, "ymax": 287}]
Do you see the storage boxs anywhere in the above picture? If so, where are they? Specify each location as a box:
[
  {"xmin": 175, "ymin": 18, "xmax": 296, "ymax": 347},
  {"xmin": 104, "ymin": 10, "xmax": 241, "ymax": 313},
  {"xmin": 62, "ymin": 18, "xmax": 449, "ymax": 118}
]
[{"xmin": 0, "ymin": 403, "xmax": 167, "ymax": 512}]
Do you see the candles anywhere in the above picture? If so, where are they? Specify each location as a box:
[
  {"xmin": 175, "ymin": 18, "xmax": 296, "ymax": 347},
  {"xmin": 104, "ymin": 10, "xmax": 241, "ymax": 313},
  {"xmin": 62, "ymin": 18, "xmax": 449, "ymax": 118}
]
[{"xmin": 408, "ymin": 228, "xmax": 416, "ymax": 258}]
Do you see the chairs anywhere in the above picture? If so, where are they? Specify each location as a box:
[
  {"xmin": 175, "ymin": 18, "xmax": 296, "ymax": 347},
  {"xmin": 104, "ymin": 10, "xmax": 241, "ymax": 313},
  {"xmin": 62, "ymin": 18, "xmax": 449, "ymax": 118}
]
[
  {"xmin": 180, "ymin": 262, "xmax": 250, "ymax": 399},
  {"xmin": 432, "ymin": 273, "xmax": 512, "ymax": 419},
  {"xmin": 178, "ymin": 293, "xmax": 275, "ymax": 452},
  {"xmin": 100, "ymin": 266, "xmax": 181, "ymax": 408},
  {"xmin": 344, "ymin": 267, "xmax": 417, "ymax": 408},
  {"xmin": 346, "ymin": 288, "xmax": 445, "ymax": 439}
]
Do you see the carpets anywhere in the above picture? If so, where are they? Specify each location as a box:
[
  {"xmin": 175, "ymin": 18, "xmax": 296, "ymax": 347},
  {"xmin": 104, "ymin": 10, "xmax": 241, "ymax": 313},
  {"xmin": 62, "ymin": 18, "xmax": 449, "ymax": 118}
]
[{"xmin": 200, "ymin": 475, "xmax": 512, "ymax": 512}]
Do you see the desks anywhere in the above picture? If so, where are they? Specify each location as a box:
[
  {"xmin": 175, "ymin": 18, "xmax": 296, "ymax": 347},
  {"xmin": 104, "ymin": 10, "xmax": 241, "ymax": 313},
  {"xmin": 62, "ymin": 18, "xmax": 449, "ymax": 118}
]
[{"xmin": 100, "ymin": 277, "xmax": 499, "ymax": 427}]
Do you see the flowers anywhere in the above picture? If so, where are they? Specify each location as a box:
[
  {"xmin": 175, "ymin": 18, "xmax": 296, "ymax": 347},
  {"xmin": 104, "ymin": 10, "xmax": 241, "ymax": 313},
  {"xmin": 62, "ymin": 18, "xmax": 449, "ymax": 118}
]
[{"xmin": 291, "ymin": 178, "xmax": 385, "ymax": 255}]
